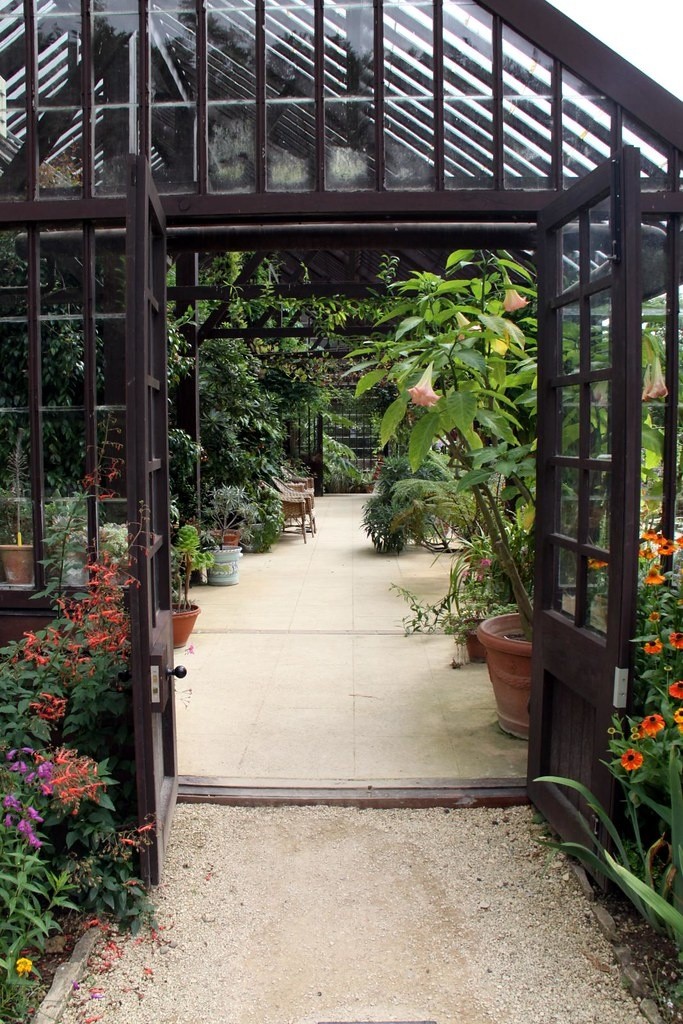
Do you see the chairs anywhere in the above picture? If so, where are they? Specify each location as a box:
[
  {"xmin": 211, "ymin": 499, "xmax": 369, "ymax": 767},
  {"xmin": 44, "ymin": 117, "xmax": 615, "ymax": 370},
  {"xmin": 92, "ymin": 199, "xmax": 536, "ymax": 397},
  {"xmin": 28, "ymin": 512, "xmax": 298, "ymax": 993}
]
[
  {"xmin": 258, "ymin": 480, "xmax": 306, "ymax": 543},
  {"xmin": 280, "ymin": 466, "xmax": 314, "ymax": 525},
  {"xmin": 271, "ymin": 475, "xmax": 316, "ymax": 538}
]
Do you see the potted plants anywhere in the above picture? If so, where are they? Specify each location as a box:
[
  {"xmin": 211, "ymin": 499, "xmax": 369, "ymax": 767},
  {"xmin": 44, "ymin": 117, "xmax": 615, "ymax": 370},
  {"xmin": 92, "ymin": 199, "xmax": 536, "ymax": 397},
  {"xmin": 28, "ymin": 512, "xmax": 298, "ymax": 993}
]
[
  {"xmin": 0, "ymin": 429, "xmax": 38, "ymax": 584},
  {"xmin": 245, "ymin": 487, "xmax": 284, "ymax": 553},
  {"xmin": 170, "ymin": 525, "xmax": 213, "ymax": 647},
  {"xmin": 205, "ymin": 485, "xmax": 257, "ymax": 586},
  {"xmin": 97, "ymin": 522, "xmax": 129, "ymax": 585},
  {"xmin": 45, "ymin": 490, "xmax": 90, "ymax": 587}
]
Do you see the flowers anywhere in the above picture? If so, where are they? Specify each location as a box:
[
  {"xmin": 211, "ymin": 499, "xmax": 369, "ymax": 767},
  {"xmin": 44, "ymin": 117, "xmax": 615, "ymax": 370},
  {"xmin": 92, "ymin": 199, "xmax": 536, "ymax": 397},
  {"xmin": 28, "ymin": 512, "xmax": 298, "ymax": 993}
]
[
  {"xmin": 0, "ymin": 406, "xmax": 159, "ymax": 1022},
  {"xmin": 444, "ymin": 560, "xmax": 519, "ymax": 643},
  {"xmin": 532, "ymin": 515, "xmax": 683, "ymax": 943},
  {"xmin": 346, "ymin": 249, "xmax": 668, "ymax": 643}
]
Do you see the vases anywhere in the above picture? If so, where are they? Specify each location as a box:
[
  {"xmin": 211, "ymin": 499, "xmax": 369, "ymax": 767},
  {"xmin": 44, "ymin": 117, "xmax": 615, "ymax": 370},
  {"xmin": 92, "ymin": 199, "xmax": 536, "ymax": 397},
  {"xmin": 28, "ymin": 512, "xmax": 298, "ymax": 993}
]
[
  {"xmin": 477, "ymin": 612, "xmax": 533, "ymax": 741},
  {"xmin": 464, "ymin": 618, "xmax": 486, "ymax": 662}
]
